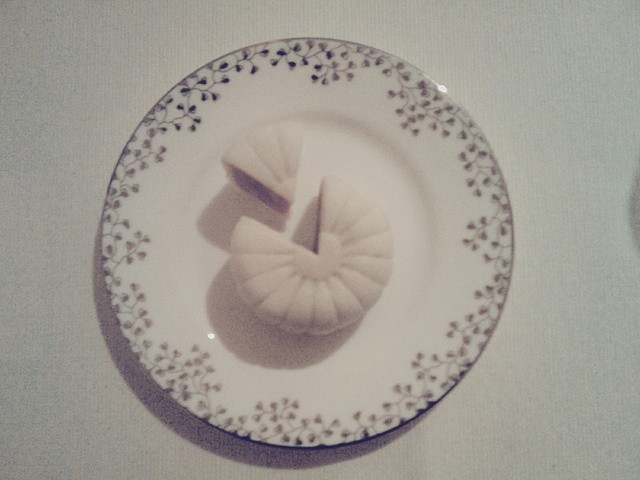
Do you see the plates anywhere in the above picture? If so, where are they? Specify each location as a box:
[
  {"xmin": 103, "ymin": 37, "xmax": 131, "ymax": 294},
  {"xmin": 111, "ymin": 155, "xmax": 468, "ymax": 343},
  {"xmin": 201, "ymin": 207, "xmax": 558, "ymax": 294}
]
[{"xmin": 100, "ymin": 37, "xmax": 513, "ymax": 450}]
